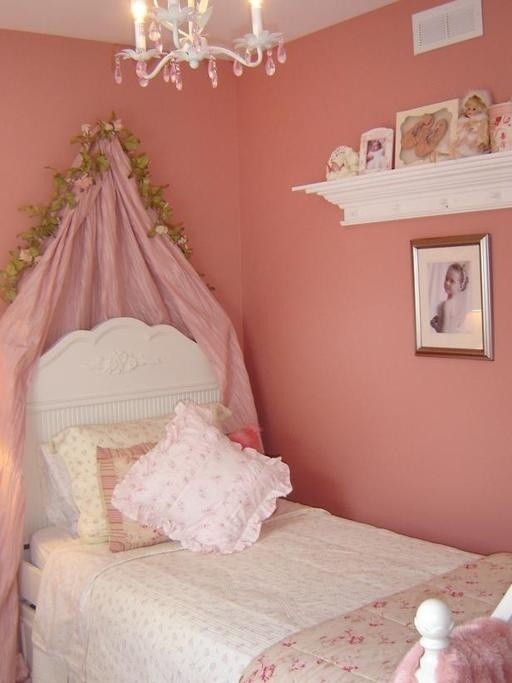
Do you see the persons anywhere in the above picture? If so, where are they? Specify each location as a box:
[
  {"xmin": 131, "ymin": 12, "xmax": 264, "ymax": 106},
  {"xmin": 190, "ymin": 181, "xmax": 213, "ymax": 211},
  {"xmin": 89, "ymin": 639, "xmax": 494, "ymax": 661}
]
[
  {"xmin": 427, "ymin": 259, "xmax": 471, "ymax": 334},
  {"xmin": 402, "ymin": 113, "xmax": 434, "ymax": 149},
  {"xmin": 415, "ymin": 118, "xmax": 448, "ymax": 157},
  {"xmin": 366, "ymin": 138, "xmax": 385, "ymax": 171},
  {"xmin": 454, "ymin": 87, "xmax": 492, "ymax": 156}
]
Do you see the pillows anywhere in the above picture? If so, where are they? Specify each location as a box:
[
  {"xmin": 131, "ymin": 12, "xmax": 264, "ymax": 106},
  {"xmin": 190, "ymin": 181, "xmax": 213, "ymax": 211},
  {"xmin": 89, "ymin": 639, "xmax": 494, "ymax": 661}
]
[
  {"xmin": 94, "ymin": 429, "xmax": 265, "ymax": 553},
  {"xmin": 41, "ymin": 402, "xmax": 240, "ymax": 543},
  {"xmin": 110, "ymin": 399, "xmax": 293, "ymax": 555}
]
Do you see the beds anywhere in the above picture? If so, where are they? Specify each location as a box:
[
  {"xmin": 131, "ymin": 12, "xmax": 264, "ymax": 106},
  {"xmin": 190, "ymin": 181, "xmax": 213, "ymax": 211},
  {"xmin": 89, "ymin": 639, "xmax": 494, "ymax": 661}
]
[{"xmin": 0, "ymin": 108, "xmax": 512, "ymax": 682}]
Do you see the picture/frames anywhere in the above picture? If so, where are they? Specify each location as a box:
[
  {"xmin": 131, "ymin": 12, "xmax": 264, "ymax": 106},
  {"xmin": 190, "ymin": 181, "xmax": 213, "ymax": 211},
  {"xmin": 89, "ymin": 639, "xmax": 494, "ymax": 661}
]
[
  {"xmin": 358, "ymin": 125, "xmax": 397, "ymax": 174},
  {"xmin": 409, "ymin": 230, "xmax": 497, "ymax": 363},
  {"xmin": 393, "ymin": 98, "xmax": 461, "ymax": 167}
]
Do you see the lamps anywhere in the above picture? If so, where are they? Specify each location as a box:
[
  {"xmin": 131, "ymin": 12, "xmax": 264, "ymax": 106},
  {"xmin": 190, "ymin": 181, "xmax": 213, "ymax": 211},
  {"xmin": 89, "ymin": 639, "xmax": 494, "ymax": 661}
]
[{"xmin": 112, "ymin": 0, "xmax": 291, "ymax": 93}]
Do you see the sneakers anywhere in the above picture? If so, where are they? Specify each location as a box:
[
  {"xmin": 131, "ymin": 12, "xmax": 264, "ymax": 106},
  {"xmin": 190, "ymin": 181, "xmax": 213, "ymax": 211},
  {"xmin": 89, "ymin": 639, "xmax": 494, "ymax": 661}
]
[{"xmin": 403, "ymin": 115, "xmax": 447, "ymax": 155}]
